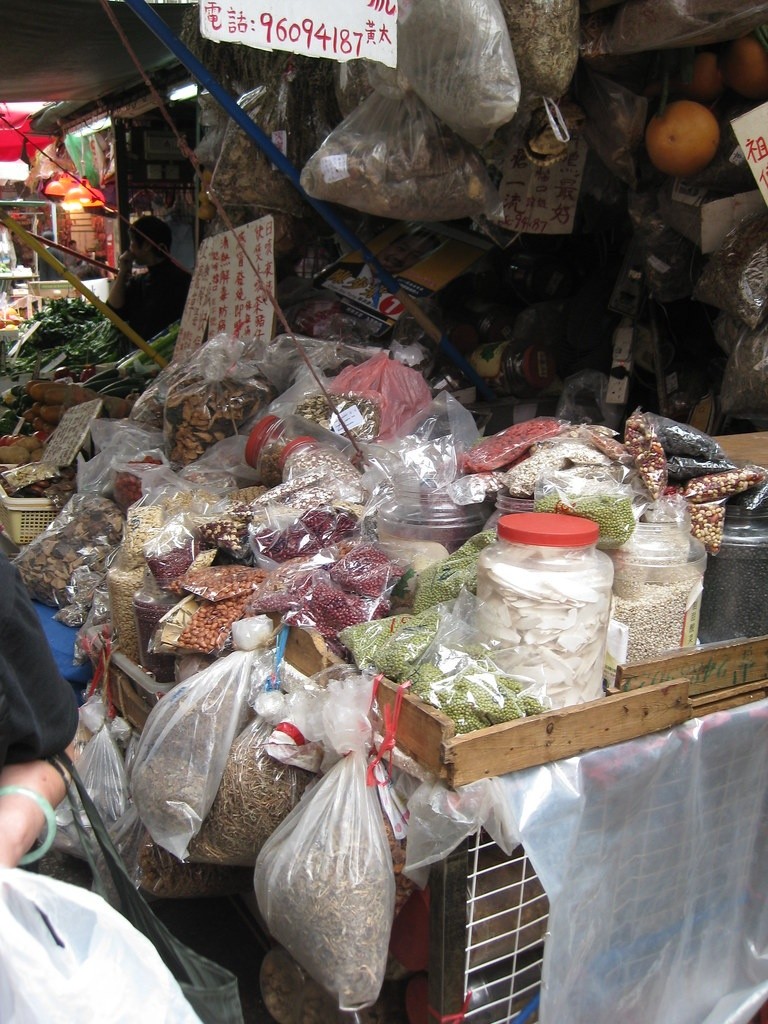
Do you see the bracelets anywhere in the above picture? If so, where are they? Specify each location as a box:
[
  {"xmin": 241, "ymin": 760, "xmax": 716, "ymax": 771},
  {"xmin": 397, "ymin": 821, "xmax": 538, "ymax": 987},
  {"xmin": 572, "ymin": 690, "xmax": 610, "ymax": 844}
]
[{"xmin": 0, "ymin": 785, "xmax": 57, "ymax": 867}]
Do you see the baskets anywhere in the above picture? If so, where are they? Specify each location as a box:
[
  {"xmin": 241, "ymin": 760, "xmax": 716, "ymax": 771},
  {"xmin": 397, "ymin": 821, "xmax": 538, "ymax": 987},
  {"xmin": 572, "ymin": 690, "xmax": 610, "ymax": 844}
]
[{"xmin": 0, "ymin": 464, "xmax": 63, "ymax": 544}]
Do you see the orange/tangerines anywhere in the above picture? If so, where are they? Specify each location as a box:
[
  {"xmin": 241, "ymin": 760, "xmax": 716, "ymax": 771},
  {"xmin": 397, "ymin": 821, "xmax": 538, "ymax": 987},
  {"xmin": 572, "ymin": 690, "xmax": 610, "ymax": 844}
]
[
  {"xmin": 684, "ymin": 52, "xmax": 722, "ymax": 97},
  {"xmin": 0, "ymin": 384, "xmax": 35, "ymax": 433},
  {"xmin": 646, "ymin": 100, "xmax": 718, "ymax": 174},
  {"xmin": 727, "ymin": 38, "xmax": 767, "ymax": 96}
]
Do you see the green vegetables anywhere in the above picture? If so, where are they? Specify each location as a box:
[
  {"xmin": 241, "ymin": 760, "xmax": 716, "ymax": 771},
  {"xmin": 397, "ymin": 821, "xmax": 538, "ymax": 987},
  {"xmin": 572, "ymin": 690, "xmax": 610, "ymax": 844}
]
[{"xmin": 10, "ymin": 297, "xmax": 122, "ymax": 373}]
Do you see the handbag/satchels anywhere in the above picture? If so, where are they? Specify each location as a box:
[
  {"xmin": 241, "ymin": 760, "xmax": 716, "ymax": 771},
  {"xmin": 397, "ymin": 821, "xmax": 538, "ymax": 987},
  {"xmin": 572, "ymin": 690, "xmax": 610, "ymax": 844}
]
[{"xmin": 0, "ymin": 748, "xmax": 242, "ymax": 1024}]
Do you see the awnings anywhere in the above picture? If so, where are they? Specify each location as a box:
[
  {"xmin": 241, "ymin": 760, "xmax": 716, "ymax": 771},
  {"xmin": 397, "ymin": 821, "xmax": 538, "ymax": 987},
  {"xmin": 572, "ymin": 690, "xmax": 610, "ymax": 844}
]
[
  {"xmin": 0, "ymin": 0, "xmax": 198, "ymax": 105},
  {"xmin": 0, "ymin": 110, "xmax": 58, "ymax": 166}
]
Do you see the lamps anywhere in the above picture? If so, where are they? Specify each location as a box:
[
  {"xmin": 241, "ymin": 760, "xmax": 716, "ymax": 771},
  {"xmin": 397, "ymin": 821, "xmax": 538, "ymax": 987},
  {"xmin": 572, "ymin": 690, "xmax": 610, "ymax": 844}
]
[
  {"xmin": 45, "ymin": 169, "xmax": 76, "ymax": 195},
  {"xmin": 66, "ymin": 176, "xmax": 105, "ymax": 206}
]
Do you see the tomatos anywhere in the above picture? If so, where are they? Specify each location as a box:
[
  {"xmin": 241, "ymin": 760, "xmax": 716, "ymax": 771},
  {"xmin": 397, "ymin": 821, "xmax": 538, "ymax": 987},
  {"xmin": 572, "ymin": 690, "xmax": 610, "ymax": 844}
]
[{"xmin": 0, "ymin": 431, "xmax": 49, "ymax": 444}]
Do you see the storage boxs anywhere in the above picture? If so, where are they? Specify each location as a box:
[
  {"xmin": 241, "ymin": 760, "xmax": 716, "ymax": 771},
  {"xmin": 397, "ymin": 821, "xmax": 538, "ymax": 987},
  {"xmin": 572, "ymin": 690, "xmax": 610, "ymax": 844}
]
[
  {"xmin": 0, "ymin": 464, "xmax": 60, "ymax": 545},
  {"xmin": 87, "ymin": 624, "xmax": 180, "ymax": 732},
  {"xmin": 29, "ymin": 278, "xmax": 108, "ymax": 303}
]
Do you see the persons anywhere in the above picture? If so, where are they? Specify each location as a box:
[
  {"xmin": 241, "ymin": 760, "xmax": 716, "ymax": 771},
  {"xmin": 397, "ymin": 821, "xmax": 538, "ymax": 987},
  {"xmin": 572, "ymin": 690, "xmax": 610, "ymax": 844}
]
[
  {"xmin": 64, "ymin": 240, "xmax": 103, "ymax": 280},
  {"xmin": 0, "ymin": 540, "xmax": 79, "ymax": 877},
  {"xmin": 106, "ymin": 216, "xmax": 193, "ymax": 357},
  {"xmin": 314, "ymin": 227, "xmax": 445, "ymax": 312},
  {"xmin": 38, "ymin": 230, "xmax": 63, "ymax": 281},
  {"xmin": 10, "ymin": 231, "xmax": 24, "ymax": 289}
]
